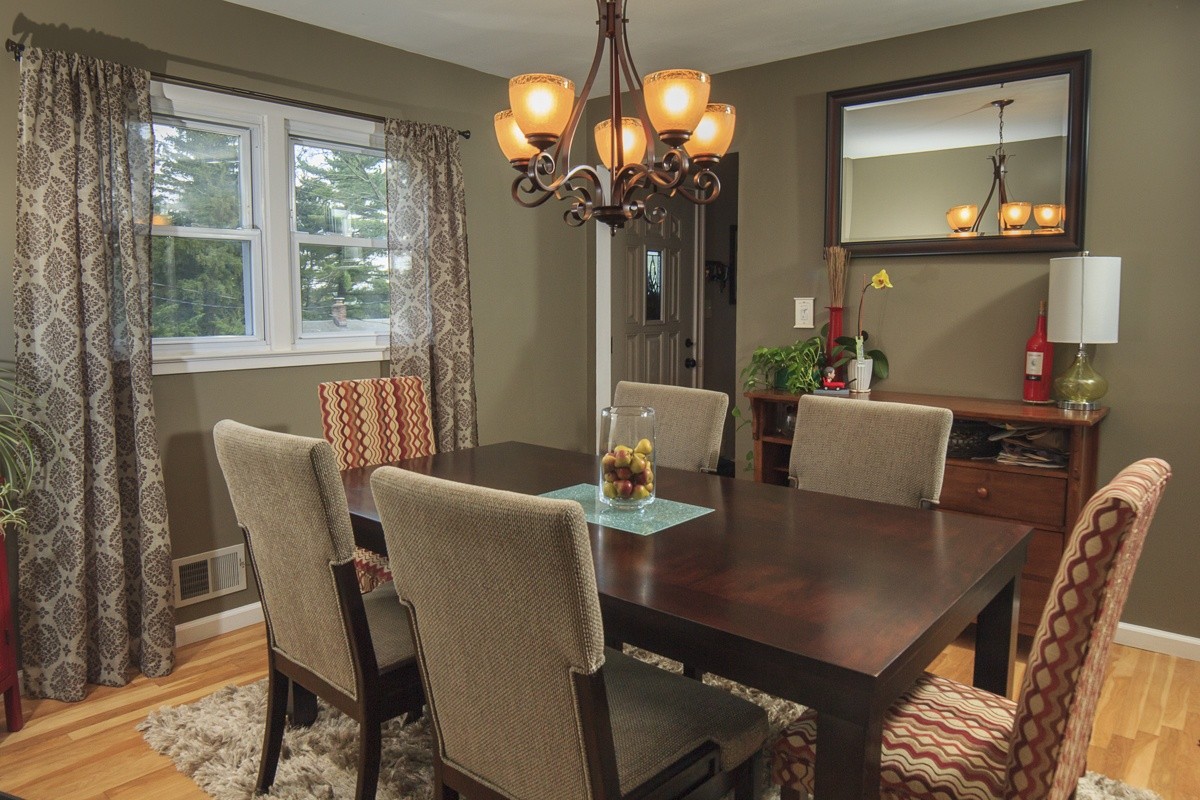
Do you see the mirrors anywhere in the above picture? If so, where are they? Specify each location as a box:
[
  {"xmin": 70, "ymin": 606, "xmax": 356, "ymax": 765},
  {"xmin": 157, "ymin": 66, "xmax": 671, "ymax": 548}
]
[{"xmin": 823, "ymin": 49, "xmax": 1092, "ymax": 261}]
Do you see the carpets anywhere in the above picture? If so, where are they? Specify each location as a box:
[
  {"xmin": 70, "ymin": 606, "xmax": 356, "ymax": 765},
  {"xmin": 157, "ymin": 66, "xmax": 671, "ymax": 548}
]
[{"xmin": 136, "ymin": 642, "xmax": 1161, "ymax": 800}]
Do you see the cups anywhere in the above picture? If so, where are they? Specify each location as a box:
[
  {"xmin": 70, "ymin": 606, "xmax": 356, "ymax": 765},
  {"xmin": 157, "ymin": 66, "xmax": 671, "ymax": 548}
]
[{"xmin": 599, "ymin": 406, "xmax": 656, "ymax": 510}]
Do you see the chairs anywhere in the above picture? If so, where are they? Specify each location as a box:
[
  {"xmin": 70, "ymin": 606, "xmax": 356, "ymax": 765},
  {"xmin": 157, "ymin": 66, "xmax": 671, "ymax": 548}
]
[
  {"xmin": 605, "ymin": 380, "xmax": 730, "ymax": 652},
  {"xmin": 317, "ymin": 374, "xmax": 436, "ymax": 594},
  {"xmin": 212, "ymin": 419, "xmax": 429, "ymax": 800},
  {"xmin": 684, "ymin": 394, "xmax": 953, "ymax": 684},
  {"xmin": 774, "ymin": 458, "xmax": 1172, "ymax": 800},
  {"xmin": 370, "ymin": 465, "xmax": 770, "ymax": 800}
]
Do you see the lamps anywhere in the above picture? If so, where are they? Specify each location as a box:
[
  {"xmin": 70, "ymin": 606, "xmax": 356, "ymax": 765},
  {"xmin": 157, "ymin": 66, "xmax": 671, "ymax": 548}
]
[
  {"xmin": 494, "ymin": 0, "xmax": 737, "ymax": 236},
  {"xmin": 1046, "ymin": 251, "xmax": 1123, "ymax": 410},
  {"xmin": 948, "ymin": 98, "xmax": 1065, "ymax": 237}
]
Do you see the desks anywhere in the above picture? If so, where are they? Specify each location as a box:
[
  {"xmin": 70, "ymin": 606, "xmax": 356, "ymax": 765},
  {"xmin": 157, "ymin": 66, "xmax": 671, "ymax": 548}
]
[{"xmin": 287, "ymin": 442, "xmax": 1035, "ymax": 800}]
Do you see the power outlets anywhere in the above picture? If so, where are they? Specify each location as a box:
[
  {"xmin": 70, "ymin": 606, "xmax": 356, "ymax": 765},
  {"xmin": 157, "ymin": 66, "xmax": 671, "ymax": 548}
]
[{"xmin": 793, "ymin": 297, "xmax": 816, "ymax": 329}]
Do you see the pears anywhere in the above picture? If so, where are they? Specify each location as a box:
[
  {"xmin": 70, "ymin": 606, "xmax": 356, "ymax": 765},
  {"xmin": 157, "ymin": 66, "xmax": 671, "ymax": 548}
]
[{"xmin": 600, "ymin": 438, "xmax": 656, "ymax": 500}]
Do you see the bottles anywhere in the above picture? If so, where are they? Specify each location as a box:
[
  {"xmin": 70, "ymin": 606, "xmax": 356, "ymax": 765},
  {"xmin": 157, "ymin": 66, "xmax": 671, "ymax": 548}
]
[
  {"xmin": 1023, "ymin": 300, "xmax": 1054, "ymax": 400},
  {"xmin": 332, "ymin": 297, "xmax": 346, "ymax": 327},
  {"xmin": 782, "ymin": 407, "xmax": 796, "ymax": 439}
]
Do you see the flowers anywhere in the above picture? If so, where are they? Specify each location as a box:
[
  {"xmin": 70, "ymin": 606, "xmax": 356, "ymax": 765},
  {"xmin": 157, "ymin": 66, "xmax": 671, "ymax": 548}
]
[{"xmin": 858, "ymin": 270, "xmax": 894, "ymax": 341}]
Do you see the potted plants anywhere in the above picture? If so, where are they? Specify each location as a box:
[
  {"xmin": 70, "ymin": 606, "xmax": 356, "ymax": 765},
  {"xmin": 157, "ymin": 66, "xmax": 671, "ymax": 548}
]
[{"xmin": 732, "ymin": 337, "xmax": 826, "ymax": 471}]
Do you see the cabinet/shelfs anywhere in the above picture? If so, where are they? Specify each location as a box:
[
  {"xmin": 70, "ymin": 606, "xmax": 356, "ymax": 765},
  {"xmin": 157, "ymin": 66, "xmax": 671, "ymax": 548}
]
[{"xmin": 742, "ymin": 390, "xmax": 1111, "ymax": 640}]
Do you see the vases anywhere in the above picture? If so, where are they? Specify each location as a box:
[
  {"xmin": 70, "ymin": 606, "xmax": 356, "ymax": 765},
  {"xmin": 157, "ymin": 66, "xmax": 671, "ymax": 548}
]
[
  {"xmin": 848, "ymin": 335, "xmax": 874, "ymax": 392},
  {"xmin": 823, "ymin": 308, "xmax": 845, "ymax": 389}
]
